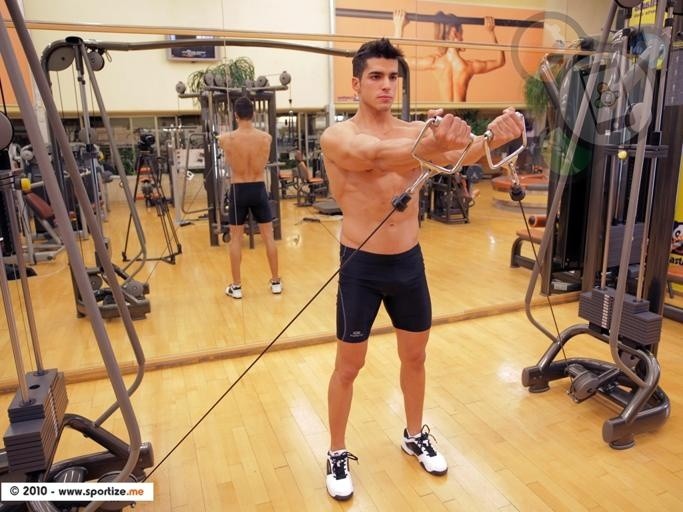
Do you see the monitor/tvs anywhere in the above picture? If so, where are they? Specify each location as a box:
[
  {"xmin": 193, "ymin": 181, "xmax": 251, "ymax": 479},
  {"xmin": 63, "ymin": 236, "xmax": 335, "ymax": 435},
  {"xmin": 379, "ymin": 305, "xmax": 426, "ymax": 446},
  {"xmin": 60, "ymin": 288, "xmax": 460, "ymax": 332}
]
[{"xmin": 166, "ymin": 35, "xmax": 219, "ymax": 61}]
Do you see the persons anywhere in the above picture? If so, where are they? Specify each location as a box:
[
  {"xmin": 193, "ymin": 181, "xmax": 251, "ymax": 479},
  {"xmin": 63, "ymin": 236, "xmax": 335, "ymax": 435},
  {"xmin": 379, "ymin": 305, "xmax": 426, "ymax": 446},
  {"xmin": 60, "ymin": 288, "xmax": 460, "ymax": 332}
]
[
  {"xmin": 319, "ymin": 38, "xmax": 525, "ymax": 500},
  {"xmin": 216, "ymin": 95, "xmax": 283, "ymax": 300},
  {"xmin": 392, "ymin": 8, "xmax": 506, "ymax": 102}
]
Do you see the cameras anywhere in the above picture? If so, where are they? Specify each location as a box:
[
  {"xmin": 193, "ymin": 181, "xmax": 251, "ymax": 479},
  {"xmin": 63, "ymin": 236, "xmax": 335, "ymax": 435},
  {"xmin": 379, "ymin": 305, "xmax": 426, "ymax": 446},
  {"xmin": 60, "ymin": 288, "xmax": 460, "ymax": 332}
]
[
  {"xmin": 121, "ymin": 150, "xmax": 183, "ymax": 263},
  {"xmin": 136, "ymin": 128, "xmax": 156, "ymax": 152}
]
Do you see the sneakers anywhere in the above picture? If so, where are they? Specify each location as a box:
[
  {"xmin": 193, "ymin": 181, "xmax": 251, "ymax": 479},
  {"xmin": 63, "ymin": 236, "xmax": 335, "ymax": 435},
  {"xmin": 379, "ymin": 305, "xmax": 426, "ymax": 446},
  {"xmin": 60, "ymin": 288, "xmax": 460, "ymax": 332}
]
[
  {"xmin": 324, "ymin": 450, "xmax": 355, "ymax": 502},
  {"xmin": 270, "ymin": 281, "xmax": 282, "ymax": 293},
  {"xmin": 225, "ymin": 284, "xmax": 243, "ymax": 299},
  {"xmin": 401, "ymin": 429, "xmax": 448, "ymax": 476}
]
